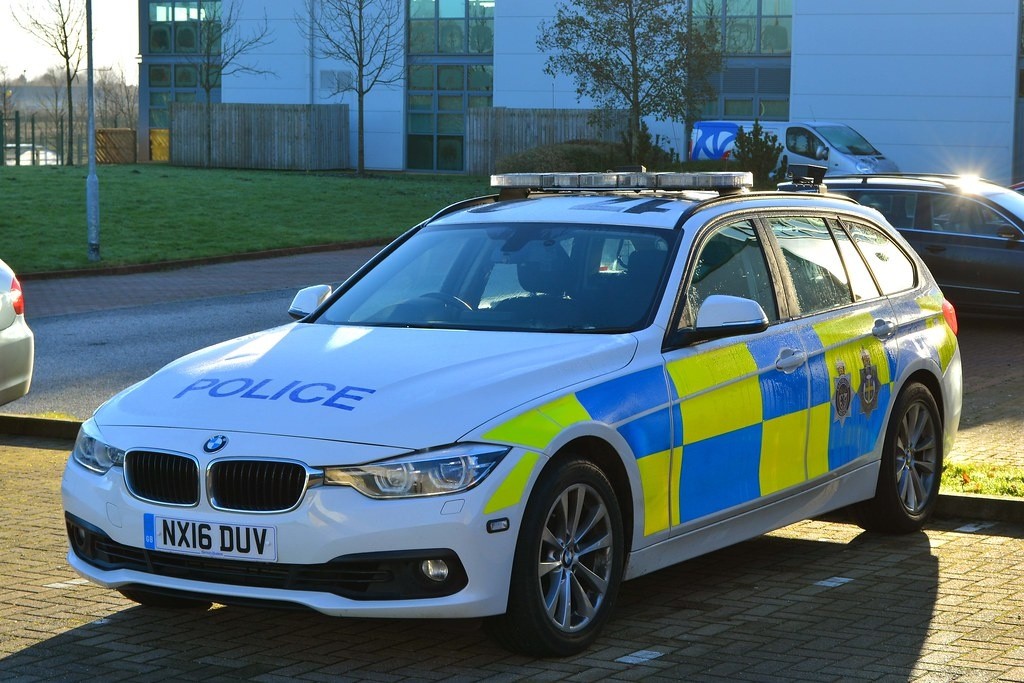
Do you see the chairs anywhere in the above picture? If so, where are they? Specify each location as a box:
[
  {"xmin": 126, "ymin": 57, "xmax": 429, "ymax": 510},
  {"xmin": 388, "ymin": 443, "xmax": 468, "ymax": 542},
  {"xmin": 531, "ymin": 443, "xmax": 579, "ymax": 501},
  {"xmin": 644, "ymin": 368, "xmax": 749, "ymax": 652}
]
[
  {"xmin": 692, "ymin": 240, "xmax": 751, "ymax": 310},
  {"xmin": 491, "ymin": 237, "xmax": 671, "ymax": 330}
]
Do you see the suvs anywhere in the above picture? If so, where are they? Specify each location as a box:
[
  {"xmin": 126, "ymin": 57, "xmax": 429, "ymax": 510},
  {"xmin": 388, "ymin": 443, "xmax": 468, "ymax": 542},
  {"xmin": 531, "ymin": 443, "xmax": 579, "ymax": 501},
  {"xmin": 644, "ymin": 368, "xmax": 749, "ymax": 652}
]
[{"xmin": 771, "ymin": 173, "xmax": 1024, "ymax": 318}]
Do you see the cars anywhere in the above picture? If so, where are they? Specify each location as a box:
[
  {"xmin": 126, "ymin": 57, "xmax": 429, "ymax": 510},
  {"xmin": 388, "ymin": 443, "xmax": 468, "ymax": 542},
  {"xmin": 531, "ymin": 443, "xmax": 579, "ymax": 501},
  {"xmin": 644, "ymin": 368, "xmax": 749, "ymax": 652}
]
[
  {"xmin": 59, "ymin": 169, "xmax": 963, "ymax": 659},
  {"xmin": 5, "ymin": 142, "xmax": 63, "ymax": 168},
  {"xmin": 0, "ymin": 258, "xmax": 35, "ymax": 410}
]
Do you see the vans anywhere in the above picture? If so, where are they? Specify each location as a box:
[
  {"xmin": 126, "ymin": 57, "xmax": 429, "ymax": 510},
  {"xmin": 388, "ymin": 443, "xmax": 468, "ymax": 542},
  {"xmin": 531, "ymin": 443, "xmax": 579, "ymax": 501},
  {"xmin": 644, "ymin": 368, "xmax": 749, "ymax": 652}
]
[{"xmin": 688, "ymin": 119, "xmax": 902, "ymax": 177}]
